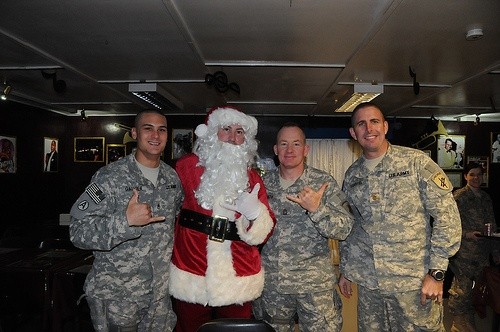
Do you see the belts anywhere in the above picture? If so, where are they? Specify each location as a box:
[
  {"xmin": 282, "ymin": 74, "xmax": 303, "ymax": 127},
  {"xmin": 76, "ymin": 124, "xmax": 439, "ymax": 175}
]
[{"xmin": 178, "ymin": 208, "xmax": 241, "ymax": 242}]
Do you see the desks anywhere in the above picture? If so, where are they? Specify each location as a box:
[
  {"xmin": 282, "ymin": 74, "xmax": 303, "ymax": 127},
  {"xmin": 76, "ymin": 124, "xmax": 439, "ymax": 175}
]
[{"xmin": 0, "ymin": 248, "xmax": 94, "ymax": 332}]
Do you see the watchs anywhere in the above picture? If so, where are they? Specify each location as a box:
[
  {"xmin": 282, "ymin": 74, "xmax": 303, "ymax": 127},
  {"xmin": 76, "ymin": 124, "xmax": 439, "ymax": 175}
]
[{"xmin": 428, "ymin": 269, "xmax": 445, "ymax": 281}]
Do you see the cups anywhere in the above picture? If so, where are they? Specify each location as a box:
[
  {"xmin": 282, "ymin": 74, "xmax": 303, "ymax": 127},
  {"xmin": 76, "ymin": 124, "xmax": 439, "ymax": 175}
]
[{"xmin": 484, "ymin": 222, "xmax": 492, "ymax": 236}]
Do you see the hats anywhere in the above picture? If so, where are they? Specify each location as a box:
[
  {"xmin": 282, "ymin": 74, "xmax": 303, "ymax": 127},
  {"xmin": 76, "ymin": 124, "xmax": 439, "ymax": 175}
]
[{"xmin": 195, "ymin": 106, "xmax": 258, "ymax": 142}]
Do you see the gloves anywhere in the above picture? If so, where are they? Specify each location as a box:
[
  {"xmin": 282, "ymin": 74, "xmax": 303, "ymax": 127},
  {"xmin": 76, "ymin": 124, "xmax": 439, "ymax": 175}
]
[{"xmin": 219, "ymin": 184, "xmax": 260, "ymax": 221}]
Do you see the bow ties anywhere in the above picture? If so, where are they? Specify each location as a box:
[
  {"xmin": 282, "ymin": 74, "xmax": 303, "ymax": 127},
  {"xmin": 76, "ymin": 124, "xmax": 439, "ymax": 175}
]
[{"xmin": 446, "ymin": 150, "xmax": 451, "ymax": 153}]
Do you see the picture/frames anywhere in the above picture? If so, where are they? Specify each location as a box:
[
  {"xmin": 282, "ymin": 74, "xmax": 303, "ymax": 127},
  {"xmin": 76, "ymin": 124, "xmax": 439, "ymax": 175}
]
[
  {"xmin": 39, "ymin": 133, "xmax": 61, "ymax": 176},
  {"xmin": 171, "ymin": 128, "xmax": 193, "ymax": 160},
  {"xmin": 0, "ymin": 134, "xmax": 17, "ymax": 176},
  {"xmin": 73, "ymin": 136, "xmax": 105, "ymax": 162},
  {"xmin": 105, "ymin": 144, "xmax": 126, "ymax": 166}
]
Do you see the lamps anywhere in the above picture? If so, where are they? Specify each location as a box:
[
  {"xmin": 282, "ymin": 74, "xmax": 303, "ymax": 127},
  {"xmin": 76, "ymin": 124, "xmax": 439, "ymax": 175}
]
[
  {"xmin": 3, "ymin": 86, "xmax": 77, "ymax": 114},
  {"xmin": 332, "ymin": 83, "xmax": 385, "ymax": 112},
  {"xmin": 128, "ymin": 83, "xmax": 184, "ymax": 113}
]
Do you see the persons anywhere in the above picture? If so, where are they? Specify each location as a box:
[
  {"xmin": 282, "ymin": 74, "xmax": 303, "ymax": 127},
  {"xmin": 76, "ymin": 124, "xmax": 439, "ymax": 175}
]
[
  {"xmin": 168, "ymin": 105, "xmax": 276, "ymax": 332},
  {"xmin": 338, "ymin": 103, "xmax": 462, "ymax": 332},
  {"xmin": 45, "ymin": 141, "xmax": 57, "ymax": 171},
  {"xmin": 442, "ymin": 138, "xmax": 456, "ymax": 167},
  {"xmin": 68, "ymin": 106, "xmax": 178, "ymax": 332},
  {"xmin": 448, "ymin": 162, "xmax": 497, "ymax": 332},
  {"xmin": 251, "ymin": 122, "xmax": 356, "ymax": 332}
]
